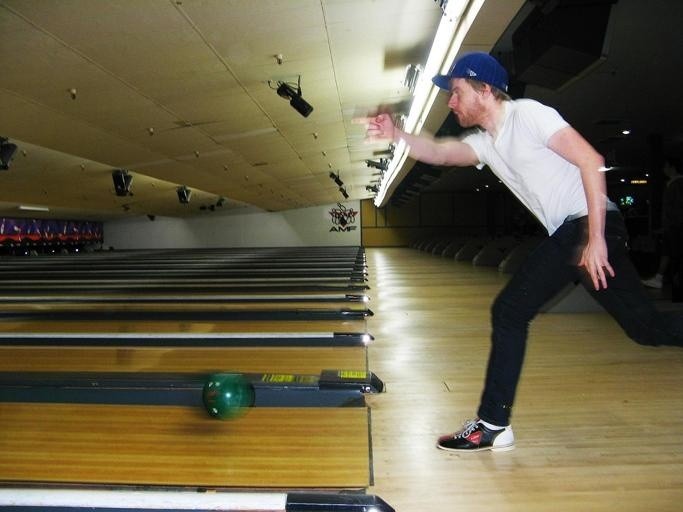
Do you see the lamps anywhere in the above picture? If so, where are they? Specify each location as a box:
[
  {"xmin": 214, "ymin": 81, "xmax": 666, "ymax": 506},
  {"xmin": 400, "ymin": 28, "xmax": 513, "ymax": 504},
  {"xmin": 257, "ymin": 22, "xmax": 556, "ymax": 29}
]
[
  {"xmin": 277, "ymin": 77, "xmax": 313, "ymax": 118},
  {"xmin": 1, "ymin": 137, "xmax": 18, "ymax": 171},
  {"xmin": 112, "ymin": 167, "xmax": 134, "ymax": 196},
  {"xmin": 178, "ymin": 187, "xmax": 194, "ymax": 203},
  {"xmin": 340, "ymin": 186, "xmax": 349, "ymax": 200},
  {"xmin": 330, "ymin": 172, "xmax": 343, "ymax": 186}
]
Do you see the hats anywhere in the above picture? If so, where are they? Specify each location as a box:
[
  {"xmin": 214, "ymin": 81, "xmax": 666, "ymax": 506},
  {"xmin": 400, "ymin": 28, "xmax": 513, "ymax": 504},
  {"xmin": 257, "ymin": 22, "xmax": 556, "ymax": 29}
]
[{"xmin": 430, "ymin": 52, "xmax": 510, "ymax": 98}]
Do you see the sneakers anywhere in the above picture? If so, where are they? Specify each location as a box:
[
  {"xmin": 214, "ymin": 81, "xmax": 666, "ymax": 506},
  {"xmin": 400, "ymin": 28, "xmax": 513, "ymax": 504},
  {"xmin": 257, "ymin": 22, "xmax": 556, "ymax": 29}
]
[{"xmin": 437, "ymin": 418, "xmax": 515, "ymax": 453}]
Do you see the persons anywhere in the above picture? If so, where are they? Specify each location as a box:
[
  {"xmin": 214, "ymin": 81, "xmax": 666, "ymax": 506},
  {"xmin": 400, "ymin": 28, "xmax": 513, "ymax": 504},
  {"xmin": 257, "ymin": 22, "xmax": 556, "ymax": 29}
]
[
  {"xmin": 346, "ymin": 52, "xmax": 682, "ymax": 454},
  {"xmin": 639, "ymin": 155, "xmax": 683, "ymax": 289}
]
